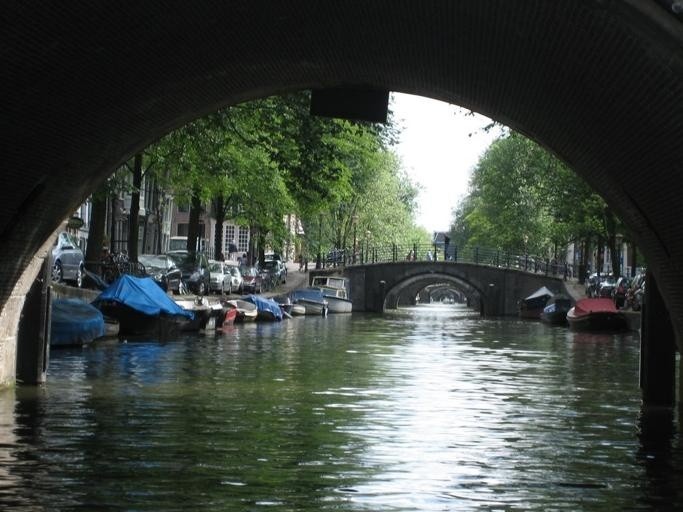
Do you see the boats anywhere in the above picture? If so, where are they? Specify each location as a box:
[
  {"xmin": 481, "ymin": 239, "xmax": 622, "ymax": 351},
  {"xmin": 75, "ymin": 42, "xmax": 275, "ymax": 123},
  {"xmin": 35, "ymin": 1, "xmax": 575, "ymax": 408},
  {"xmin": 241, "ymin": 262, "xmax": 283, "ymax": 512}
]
[
  {"xmin": 520, "ymin": 286, "xmax": 623, "ymax": 335},
  {"xmin": 93, "ymin": 275, "xmax": 354, "ymax": 343}
]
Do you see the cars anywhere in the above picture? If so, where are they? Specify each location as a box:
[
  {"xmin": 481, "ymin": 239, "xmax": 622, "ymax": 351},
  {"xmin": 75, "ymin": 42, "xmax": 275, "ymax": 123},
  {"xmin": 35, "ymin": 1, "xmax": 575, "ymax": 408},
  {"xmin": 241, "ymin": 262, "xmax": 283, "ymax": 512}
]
[
  {"xmin": 511, "ymin": 255, "xmax": 571, "ymax": 278},
  {"xmin": 135, "ymin": 248, "xmax": 288, "ymax": 295},
  {"xmin": 586, "ymin": 267, "xmax": 646, "ymax": 311},
  {"xmin": 49, "ymin": 232, "xmax": 86, "ymax": 288}
]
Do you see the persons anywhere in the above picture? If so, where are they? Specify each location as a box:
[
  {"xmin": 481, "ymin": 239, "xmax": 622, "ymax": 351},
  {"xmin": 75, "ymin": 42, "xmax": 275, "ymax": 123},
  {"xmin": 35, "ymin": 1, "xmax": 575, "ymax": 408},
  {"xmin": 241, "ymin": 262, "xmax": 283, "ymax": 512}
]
[
  {"xmin": 304, "ymin": 253, "xmax": 308, "ymax": 272},
  {"xmin": 298, "ymin": 254, "xmax": 302, "ymax": 271},
  {"xmin": 551, "ymin": 255, "xmax": 559, "ymax": 277},
  {"xmin": 406, "ymin": 248, "xmax": 453, "ymax": 262},
  {"xmin": 567, "ymin": 261, "xmax": 592, "ymax": 281}
]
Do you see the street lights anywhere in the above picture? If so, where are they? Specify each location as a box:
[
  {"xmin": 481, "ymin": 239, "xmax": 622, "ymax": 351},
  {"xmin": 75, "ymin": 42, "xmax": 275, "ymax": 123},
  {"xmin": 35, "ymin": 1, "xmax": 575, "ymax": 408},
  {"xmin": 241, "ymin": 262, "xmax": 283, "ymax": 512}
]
[
  {"xmin": 522, "ymin": 232, "xmax": 529, "ymax": 271},
  {"xmin": 352, "ymin": 215, "xmax": 359, "ymax": 264},
  {"xmin": 315, "ymin": 210, "xmax": 327, "ymax": 269},
  {"xmin": 365, "ymin": 230, "xmax": 371, "ymax": 263}
]
